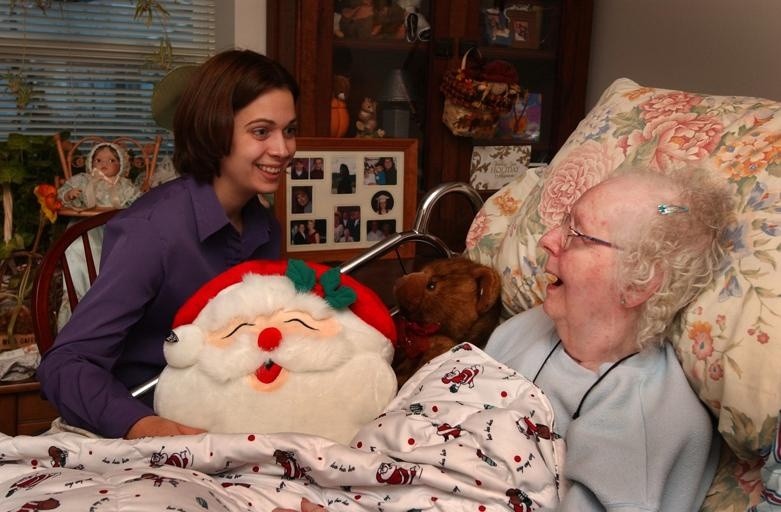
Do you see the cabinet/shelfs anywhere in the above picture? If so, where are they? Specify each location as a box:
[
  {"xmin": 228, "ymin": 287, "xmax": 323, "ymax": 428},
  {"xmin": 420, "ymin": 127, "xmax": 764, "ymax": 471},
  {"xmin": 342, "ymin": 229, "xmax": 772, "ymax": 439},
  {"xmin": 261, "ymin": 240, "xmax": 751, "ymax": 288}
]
[{"xmin": 265, "ymin": 0, "xmax": 593, "ymax": 310}]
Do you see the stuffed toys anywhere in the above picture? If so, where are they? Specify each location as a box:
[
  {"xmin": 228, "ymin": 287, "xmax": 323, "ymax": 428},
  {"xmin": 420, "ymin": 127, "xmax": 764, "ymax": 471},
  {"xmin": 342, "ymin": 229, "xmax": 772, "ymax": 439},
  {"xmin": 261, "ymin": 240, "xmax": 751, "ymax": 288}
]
[
  {"xmin": 392, "ymin": 255, "xmax": 500, "ymax": 390},
  {"xmin": 331, "ymin": 76, "xmax": 351, "ymax": 101},
  {"xmin": 154, "ymin": 259, "xmax": 399, "ymax": 445},
  {"xmin": 356, "ymin": 96, "xmax": 377, "ymax": 138}
]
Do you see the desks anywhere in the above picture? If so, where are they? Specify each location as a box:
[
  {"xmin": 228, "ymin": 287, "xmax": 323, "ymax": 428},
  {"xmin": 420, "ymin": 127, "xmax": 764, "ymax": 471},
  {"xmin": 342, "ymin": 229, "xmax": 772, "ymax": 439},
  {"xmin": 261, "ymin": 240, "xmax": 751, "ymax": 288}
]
[{"xmin": 0, "ymin": 375, "xmax": 63, "ymax": 436}]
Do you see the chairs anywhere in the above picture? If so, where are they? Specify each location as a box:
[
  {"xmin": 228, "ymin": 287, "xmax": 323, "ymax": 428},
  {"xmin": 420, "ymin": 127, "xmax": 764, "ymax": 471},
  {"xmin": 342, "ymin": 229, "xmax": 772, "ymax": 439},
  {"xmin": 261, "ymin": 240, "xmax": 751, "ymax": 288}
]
[{"xmin": 30, "ymin": 208, "xmax": 127, "ymax": 357}]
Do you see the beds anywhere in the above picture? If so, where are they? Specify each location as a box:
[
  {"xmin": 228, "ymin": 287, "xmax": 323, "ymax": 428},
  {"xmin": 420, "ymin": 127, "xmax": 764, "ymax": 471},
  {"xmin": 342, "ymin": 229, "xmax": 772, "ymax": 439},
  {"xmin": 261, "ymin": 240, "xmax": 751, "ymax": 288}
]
[{"xmin": 0, "ymin": 184, "xmax": 677, "ymax": 512}]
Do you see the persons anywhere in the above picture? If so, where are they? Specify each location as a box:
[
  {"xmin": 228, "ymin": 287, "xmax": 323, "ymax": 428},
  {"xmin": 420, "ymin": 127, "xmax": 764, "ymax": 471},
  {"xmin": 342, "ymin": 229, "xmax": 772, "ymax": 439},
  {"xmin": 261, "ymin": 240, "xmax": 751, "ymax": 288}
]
[
  {"xmin": 290, "ymin": 156, "xmax": 396, "ymax": 243},
  {"xmin": 37, "ymin": 49, "xmax": 296, "ymax": 437},
  {"xmin": 56, "ymin": 142, "xmax": 144, "ymax": 209},
  {"xmin": 485, "ymin": 167, "xmax": 735, "ymax": 512}
]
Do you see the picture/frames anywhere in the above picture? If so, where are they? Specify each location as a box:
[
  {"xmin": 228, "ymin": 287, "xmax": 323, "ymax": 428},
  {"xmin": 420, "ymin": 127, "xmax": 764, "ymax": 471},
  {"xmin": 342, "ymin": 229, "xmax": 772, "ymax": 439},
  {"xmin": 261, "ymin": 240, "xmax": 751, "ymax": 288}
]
[{"xmin": 274, "ymin": 138, "xmax": 418, "ymax": 264}]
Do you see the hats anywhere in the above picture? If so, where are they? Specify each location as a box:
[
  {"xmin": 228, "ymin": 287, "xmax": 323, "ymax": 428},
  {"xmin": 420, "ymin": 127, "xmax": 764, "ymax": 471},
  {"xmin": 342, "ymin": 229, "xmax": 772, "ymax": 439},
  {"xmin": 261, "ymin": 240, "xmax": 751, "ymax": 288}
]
[{"xmin": 375, "ymin": 195, "xmax": 389, "ymax": 208}]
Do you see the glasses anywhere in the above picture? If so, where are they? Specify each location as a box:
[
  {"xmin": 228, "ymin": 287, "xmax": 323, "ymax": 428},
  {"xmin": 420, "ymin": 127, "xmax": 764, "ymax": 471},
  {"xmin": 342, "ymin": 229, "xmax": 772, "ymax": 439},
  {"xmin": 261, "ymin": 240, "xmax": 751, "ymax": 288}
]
[{"xmin": 560, "ymin": 205, "xmax": 627, "ymax": 251}]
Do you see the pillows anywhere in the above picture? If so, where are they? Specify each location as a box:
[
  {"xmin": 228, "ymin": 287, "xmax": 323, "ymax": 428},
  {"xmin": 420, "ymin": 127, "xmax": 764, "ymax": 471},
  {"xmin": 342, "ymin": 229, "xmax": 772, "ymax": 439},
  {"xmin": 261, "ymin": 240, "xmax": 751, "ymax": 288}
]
[
  {"xmin": 461, "ymin": 166, "xmax": 546, "ymax": 268},
  {"xmin": 492, "ymin": 77, "xmax": 781, "ymax": 470}
]
